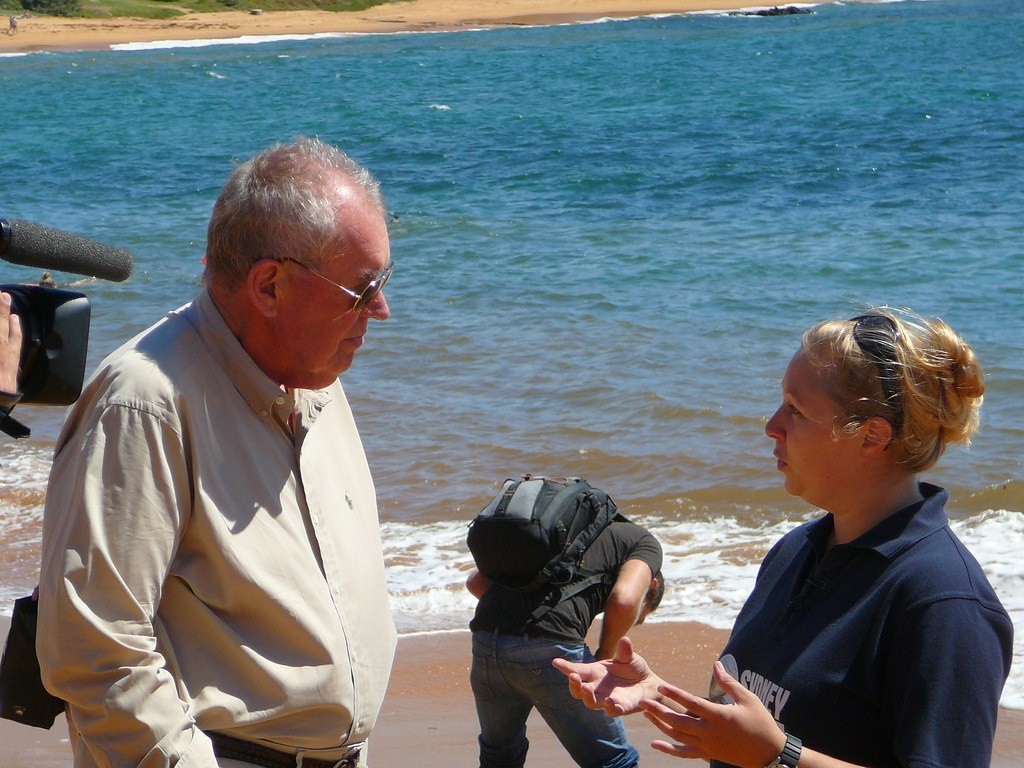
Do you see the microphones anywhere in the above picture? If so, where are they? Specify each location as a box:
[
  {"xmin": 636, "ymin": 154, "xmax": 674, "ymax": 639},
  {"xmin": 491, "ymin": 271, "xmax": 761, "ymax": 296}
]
[{"xmin": 0, "ymin": 218, "xmax": 133, "ymax": 282}]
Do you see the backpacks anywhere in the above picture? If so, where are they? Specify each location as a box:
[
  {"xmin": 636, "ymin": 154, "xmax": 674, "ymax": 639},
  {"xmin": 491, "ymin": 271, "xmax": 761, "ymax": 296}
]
[{"xmin": 465, "ymin": 472, "xmax": 636, "ymax": 621}]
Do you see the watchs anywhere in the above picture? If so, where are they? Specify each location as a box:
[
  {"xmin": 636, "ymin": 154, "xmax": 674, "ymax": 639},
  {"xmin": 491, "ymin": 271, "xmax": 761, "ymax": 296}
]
[{"xmin": 764, "ymin": 733, "xmax": 802, "ymax": 768}]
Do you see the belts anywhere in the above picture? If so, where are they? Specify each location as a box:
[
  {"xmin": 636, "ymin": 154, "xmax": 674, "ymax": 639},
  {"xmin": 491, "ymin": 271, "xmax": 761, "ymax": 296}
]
[
  {"xmin": 485, "ymin": 628, "xmax": 537, "ymax": 639},
  {"xmin": 202, "ymin": 731, "xmax": 361, "ymax": 768}
]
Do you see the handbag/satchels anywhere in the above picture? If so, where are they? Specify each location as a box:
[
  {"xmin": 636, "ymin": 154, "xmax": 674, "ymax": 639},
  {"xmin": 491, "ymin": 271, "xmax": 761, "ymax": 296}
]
[{"xmin": 0, "ymin": 586, "xmax": 66, "ymax": 730}]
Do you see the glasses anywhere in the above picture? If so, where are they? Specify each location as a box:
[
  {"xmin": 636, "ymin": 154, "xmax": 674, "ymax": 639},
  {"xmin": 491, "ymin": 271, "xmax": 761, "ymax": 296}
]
[
  {"xmin": 273, "ymin": 255, "xmax": 395, "ymax": 314},
  {"xmin": 851, "ymin": 312, "xmax": 907, "ymax": 435}
]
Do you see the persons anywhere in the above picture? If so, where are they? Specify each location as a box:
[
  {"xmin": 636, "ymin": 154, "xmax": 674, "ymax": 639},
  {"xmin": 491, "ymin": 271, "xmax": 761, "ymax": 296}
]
[
  {"xmin": 34, "ymin": 140, "xmax": 397, "ymax": 768},
  {"xmin": 553, "ymin": 310, "xmax": 1014, "ymax": 767},
  {"xmin": 465, "ymin": 474, "xmax": 664, "ymax": 767}
]
